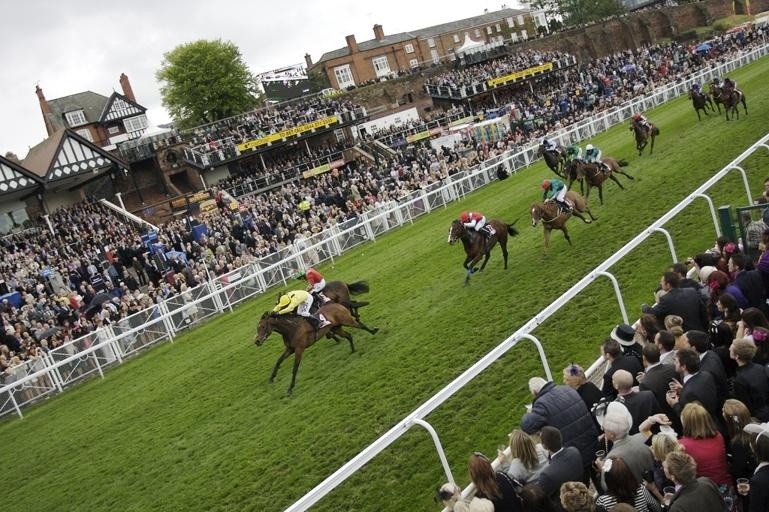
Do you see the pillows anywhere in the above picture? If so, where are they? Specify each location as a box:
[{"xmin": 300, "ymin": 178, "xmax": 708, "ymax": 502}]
[
  {"xmin": 279, "ymin": 295, "xmax": 291, "ymax": 308},
  {"xmin": 460, "ymin": 211, "xmax": 469, "ymax": 223},
  {"xmin": 585, "ymin": 144, "xmax": 593, "ymax": 150},
  {"xmin": 296, "ymin": 270, "xmax": 305, "ymax": 281},
  {"xmin": 543, "ymin": 180, "xmax": 551, "ymax": 190}
]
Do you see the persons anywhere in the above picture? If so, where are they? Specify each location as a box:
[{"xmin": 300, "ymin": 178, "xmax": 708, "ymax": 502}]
[
  {"xmin": 271, "ymin": 289, "xmax": 332, "ymax": 329},
  {"xmin": 542, "ymin": 138, "xmax": 562, "ymax": 153},
  {"xmin": 635, "ymin": 112, "xmax": 653, "ymax": 131},
  {"xmin": 567, "ymin": 145, "xmax": 587, "ymax": 163},
  {"xmin": 585, "ymin": 144, "xmax": 611, "ymax": 172},
  {"xmin": 542, "ymin": 177, "xmax": 574, "ymax": 212},
  {"xmin": 691, "ymin": 81, "xmax": 707, "ymax": 98},
  {"xmin": 0, "ymin": 69, "xmax": 456, "ymax": 414},
  {"xmin": 296, "ymin": 267, "xmax": 331, "ymax": 305},
  {"xmin": 724, "ymin": 78, "xmax": 742, "ymax": 95},
  {"xmin": 420, "ymin": 6, "xmax": 765, "ymax": 210},
  {"xmin": 459, "ymin": 210, "xmax": 493, "ymax": 240},
  {"xmin": 714, "ymin": 77, "xmax": 727, "ymax": 89},
  {"xmin": 431, "ymin": 192, "xmax": 765, "ymax": 512}
]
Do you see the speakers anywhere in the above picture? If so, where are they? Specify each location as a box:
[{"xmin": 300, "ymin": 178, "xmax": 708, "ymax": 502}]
[
  {"xmin": 314, "ymin": 295, "xmax": 325, "ymax": 307},
  {"xmin": 308, "ymin": 314, "xmax": 324, "ymax": 329}
]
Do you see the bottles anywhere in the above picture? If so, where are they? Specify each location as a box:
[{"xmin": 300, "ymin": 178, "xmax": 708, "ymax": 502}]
[
  {"xmin": 743, "ymin": 422, "xmax": 768, "ymax": 438},
  {"xmin": 591, "ymin": 402, "xmax": 633, "ymax": 433},
  {"xmin": 610, "ymin": 323, "xmax": 637, "ymax": 347}
]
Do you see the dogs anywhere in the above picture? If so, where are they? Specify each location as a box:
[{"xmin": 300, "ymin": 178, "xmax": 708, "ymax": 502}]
[
  {"xmin": 574, "ymin": 157, "xmax": 635, "ymax": 206},
  {"xmin": 629, "ymin": 117, "xmax": 660, "ymax": 156},
  {"xmin": 254, "ymin": 299, "xmax": 380, "ymax": 396},
  {"xmin": 447, "ymin": 217, "xmax": 517, "ymax": 287},
  {"xmin": 563, "ymin": 159, "xmax": 585, "ymax": 197},
  {"xmin": 529, "ymin": 190, "xmax": 599, "ymax": 259},
  {"xmin": 708, "ymin": 83, "xmax": 726, "ymax": 116},
  {"xmin": 687, "ymin": 89, "xmax": 715, "ymax": 121},
  {"xmin": 717, "ymin": 87, "xmax": 748, "ymax": 121},
  {"xmin": 537, "ymin": 143, "xmax": 570, "ymax": 180},
  {"xmin": 275, "ymin": 279, "xmax": 370, "ymax": 344}
]
